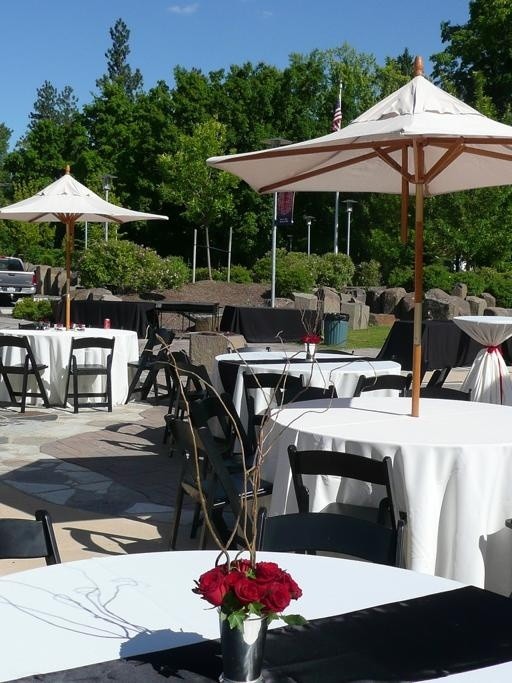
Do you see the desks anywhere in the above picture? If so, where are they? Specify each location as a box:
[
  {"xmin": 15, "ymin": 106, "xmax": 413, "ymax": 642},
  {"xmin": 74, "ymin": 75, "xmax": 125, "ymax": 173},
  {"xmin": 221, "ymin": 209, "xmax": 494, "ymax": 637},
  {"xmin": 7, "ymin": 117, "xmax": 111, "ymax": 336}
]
[
  {"xmin": 376, "ymin": 320, "xmax": 509, "ymax": 370},
  {"xmin": 50, "ymin": 300, "xmax": 220, "ymax": 339},
  {"xmin": 1, "ymin": 328, "xmax": 139, "ymax": 407},
  {"xmin": 221, "ymin": 305, "xmax": 320, "ymax": 342},
  {"xmin": 0, "ymin": 551, "xmax": 510, "ymax": 682}
]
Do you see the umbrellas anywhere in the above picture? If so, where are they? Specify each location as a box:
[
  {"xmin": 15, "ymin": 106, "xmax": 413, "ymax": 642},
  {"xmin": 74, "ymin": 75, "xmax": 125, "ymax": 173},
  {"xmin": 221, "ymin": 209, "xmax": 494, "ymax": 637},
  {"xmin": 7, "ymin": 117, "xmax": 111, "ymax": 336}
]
[
  {"xmin": 205, "ymin": 57, "xmax": 512, "ymax": 416},
  {"xmin": 0, "ymin": 164, "xmax": 170, "ymax": 331}
]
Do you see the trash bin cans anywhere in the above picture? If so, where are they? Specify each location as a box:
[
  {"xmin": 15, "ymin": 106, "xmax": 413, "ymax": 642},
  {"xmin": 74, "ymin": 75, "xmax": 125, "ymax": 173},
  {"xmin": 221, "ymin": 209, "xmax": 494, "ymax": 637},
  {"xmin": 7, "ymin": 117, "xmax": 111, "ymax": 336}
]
[{"xmin": 324, "ymin": 313, "xmax": 349, "ymax": 346}]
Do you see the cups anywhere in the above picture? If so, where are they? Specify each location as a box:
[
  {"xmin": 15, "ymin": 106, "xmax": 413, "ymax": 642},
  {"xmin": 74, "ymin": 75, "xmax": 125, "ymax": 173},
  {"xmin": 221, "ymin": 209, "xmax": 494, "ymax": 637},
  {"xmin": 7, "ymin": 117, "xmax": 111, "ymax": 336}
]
[
  {"xmin": 104, "ymin": 319, "xmax": 111, "ymax": 330},
  {"xmin": 34, "ymin": 320, "xmax": 86, "ymax": 332}
]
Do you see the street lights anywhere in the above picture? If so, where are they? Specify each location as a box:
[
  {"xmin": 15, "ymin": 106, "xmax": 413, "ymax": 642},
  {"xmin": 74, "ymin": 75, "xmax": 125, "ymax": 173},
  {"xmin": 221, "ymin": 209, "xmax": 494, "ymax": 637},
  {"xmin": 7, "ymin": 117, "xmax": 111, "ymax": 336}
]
[
  {"xmin": 302, "ymin": 214, "xmax": 315, "ymax": 257},
  {"xmin": 256, "ymin": 133, "xmax": 293, "ymax": 307},
  {"xmin": 341, "ymin": 198, "xmax": 357, "ymax": 256},
  {"xmin": 97, "ymin": 173, "xmax": 120, "ymax": 241}
]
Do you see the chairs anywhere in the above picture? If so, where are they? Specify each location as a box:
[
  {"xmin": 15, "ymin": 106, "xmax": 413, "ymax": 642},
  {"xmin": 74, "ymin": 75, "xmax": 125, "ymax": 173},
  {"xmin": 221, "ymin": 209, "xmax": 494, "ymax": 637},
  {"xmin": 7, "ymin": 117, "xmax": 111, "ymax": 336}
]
[
  {"xmin": 124, "ymin": 328, "xmax": 176, "ymax": 406},
  {"xmin": 18, "ymin": 323, "xmax": 44, "ymax": 330},
  {"xmin": 63, "ymin": 336, "xmax": 115, "ymax": 414},
  {"xmin": 1, "ymin": 336, "xmax": 50, "ymax": 412},
  {"xmin": 163, "ymin": 346, "xmax": 512, "ymax": 601},
  {"xmin": 1, "ymin": 509, "xmax": 60, "ymax": 566}
]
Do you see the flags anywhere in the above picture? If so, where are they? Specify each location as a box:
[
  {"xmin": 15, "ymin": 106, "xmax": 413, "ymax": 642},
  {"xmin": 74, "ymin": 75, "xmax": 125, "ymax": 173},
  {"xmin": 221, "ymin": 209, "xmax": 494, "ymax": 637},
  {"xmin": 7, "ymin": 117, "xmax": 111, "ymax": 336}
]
[{"xmin": 331, "ymin": 95, "xmax": 342, "ymax": 131}]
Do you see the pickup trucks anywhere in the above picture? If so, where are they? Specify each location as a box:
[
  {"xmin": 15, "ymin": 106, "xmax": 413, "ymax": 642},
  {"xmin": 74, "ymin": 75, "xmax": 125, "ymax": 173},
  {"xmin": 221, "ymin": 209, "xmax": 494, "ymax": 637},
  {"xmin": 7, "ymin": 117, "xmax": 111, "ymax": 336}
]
[{"xmin": 0, "ymin": 255, "xmax": 38, "ymax": 304}]
